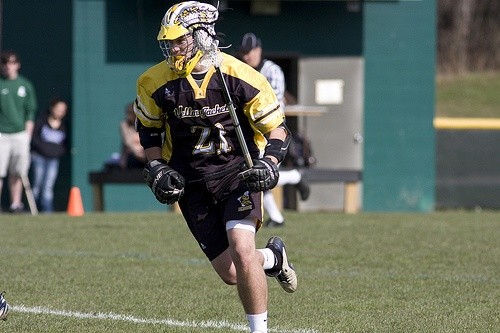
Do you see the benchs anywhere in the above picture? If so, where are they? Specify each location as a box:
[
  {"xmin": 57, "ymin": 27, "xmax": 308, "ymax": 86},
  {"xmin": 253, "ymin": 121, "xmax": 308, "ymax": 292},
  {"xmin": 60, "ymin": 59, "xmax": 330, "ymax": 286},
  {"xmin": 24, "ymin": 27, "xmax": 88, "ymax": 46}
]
[{"xmin": 87, "ymin": 170, "xmax": 363, "ymax": 215}]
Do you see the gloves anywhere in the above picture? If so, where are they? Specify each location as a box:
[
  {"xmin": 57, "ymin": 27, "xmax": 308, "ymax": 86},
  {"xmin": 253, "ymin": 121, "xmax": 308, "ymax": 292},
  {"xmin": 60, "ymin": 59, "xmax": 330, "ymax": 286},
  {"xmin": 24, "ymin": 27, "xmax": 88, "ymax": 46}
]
[
  {"xmin": 142, "ymin": 159, "xmax": 185, "ymax": 205},
  {"xmin": 237, "ymin": 157, "xmax": 280, "ymax": 192}
]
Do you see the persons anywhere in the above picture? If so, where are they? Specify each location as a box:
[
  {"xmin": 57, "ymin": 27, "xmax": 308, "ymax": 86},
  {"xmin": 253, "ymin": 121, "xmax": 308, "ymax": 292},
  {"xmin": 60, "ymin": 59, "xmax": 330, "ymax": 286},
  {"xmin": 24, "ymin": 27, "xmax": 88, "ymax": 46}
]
[
  {"xmin": 133, "ymin": 1, "xmax": 298, "ymax": 333},
  {"xmin": 27, "ymin": 98, "xmax": 69, "ymax": 212},
  {"xmin": 121, "ymin": 102, "xmax": 181, "ymax": 212},
  {"xmin": 236, "ymin": 32, "xmax": 310, "ymax": 227},
  {"xmin": 0, "ymin": 50, "xmax": 37, "ymax": 213}
]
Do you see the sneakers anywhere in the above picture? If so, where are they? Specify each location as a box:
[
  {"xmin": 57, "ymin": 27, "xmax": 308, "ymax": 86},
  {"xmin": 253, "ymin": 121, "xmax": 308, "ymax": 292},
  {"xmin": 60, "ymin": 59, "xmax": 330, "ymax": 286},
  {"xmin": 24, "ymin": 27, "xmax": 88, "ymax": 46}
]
[
  {"xmin": 264, "ymin": 236, "xmax": 297, "ymax": 293},
  {"xmin": 0, "ymin": 291, "xmax": 9, "ymax": 321},
  {"xmin": 234, "ymin": 33, "xmax": 262, "ymax": 52},
  {"xmin": 261, "ymin": 218, "xmax": 285, "ymax": 229},
  {"xmin": 297, "ymin": 168, "xmax": 310, "ymax": 200},
  {"xmin": 10, "ymin": 207, "xmax": 23, "ymax": 214}
]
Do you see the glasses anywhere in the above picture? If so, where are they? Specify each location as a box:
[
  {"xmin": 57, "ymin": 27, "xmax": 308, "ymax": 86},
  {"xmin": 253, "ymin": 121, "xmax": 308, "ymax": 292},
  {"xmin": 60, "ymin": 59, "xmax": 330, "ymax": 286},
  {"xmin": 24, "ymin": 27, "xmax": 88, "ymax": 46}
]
[{"xmin": 3, "ymin": 60, "xmax": 19, "ymax": 65}]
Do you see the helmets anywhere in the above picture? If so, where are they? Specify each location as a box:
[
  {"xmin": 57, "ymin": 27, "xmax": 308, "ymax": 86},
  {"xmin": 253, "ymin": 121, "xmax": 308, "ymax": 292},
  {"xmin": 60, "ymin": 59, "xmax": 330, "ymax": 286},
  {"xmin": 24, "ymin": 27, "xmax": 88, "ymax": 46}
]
[{"xmin": 157, "ymin": 2, "xmax": 219, "ymax": 78}]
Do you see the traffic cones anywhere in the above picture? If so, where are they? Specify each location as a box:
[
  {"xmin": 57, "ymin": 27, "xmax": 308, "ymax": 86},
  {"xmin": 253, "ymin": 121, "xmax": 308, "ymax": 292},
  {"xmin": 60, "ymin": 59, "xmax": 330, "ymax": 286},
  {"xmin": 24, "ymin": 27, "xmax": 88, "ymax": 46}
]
[{"xmin": 66, "ymin": 187, "xmax": 85, "ymax": 217}]
